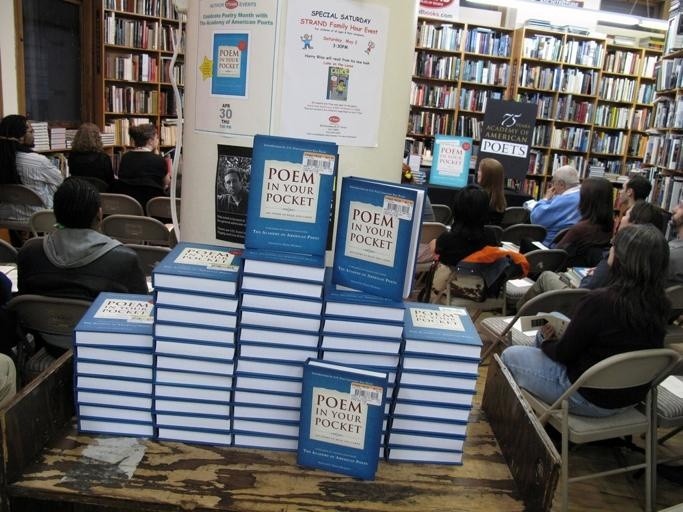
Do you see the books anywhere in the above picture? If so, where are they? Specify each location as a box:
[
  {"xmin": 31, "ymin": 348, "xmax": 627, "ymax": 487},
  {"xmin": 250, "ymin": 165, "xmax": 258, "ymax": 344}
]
[
  {"xmin": 154, "ymin": 425, "xmax": 232, "ymax": 447},
  {"xmin": 322, "ymin": 266, "xmax": 406, "ymax": 324},
  {"xmin": 527, "ymin": 149, "xmax": 587, "ymax": 180},
  {"xmin": 47, "ymin": 128, "xmax": 65, "ymax": 149},
  {"xmin": 590, "ymin": 130, "xmax": 627, "ymax": 156},
  {"xmin": 153, "ymin": 383, "xmax": 230, "ymax": 403},
  {"xmin": 151, "ymin": 288, "xmax": 239, "ymax": 315},
  {"xmin": 153, "ymin": 354, "xmax": 235, "ymax": 377},
  {"xmin": 26, "ymin": 120, "xmax": 49, "ymax": 149},
  {"xmin": 385, "ymin": 446, "xmax": 463, "ymax": 464},
  {"xmin": 603, "ymin": 51, "xmax": 641, "ymax": 76},
  {"xmin": 72, "ymin": 291, "xmax": 153, "ymax": 351},
  {"xmin": 564, "ymin": 39, "xmax": 603, "ymax": 69},
  {"xmin": 236, "ymin": 325, "xmax": 322, "ymax": 349},
  {"xmin": 151, "ymin": 322, "xmax": 236, "ymax": 347},
  {"xmin": 319, "ymin": 316, "xmax": 406, "ymax": 341},
  {"xmin": 151, "ymin": 241, "xmax": 243, "ymax": 298},
  {"xmin": 332, "ymin": 175, "xmax": 425, "ymax": 302},
  {"xmin": 237, "ymin": 273, "xmax": 324, "ymax": 301},
  {"xmin": 237, "ymin": 308, "xmax": 323, "ymax": 335},
  {"xmin": 161, "ymin": 0, "xmax": 179, "ymax": 20},
  {"xmin": 555, "ymin": 95, "xmax": 593, "ymax": 124},
  {"xmin": 628, "ymin": 133, "xmax": 648, "ymax": 157},
  {"xmin": 74, "ymin": 404, "xmax": 154, "ymax": 425},
  {"xmin": 640, "ymin": 167, "xmax": 683, "ymax": 214},
  {"xmin": 631, "ymin": 107, "xmax": 652, "ymax": 131},
  {"xmin": 599, "ymin": 76, "xmax": 635, "ymax": 103},
  {"xmin": 464, "ymin": 27, "xmax": 511, "ymax": 58},
  {"xmin": 456, "ymin": 116, "xmax": 483, "ymax": 141},
  {"xmin": 234, "ymin": 358, "xmax": 303, "ymax": 380},
  {"xmin": 407, "ymin": 111, "xmax": 453, "ymax": 134},
  {"xmin": 318, "ymin": 334, "xmax": 402, "ymax": 356},
  {"xmin": 655, "ymin": 58, "xmax": 682, "ymax": 92},
  {"xmin": 104, "ymin": 0, "xmax": 159, "ymax": 17},
  {"xmin": 518, "ymin": 63, "xmax": 561, "ymax": 91},
  {"xmin": 594, "ymin": 102, "xmax": 630, "ymax": 129},
  {"xmin": 462, "ymin": 60, "xmax": 510, "ymax": 87},
  {"xmin": 553, "ymin": 127, "xmax": 590, "ymax": 153},
  {"xmin": 65, "ymin": 130, "xmax": 78, "ymax": 149},
  {"xmin": 78, "ymin": 418, "xmax": 156, "ymax": 439},
  {"xmin": 381, "ymin": 417, "xmax": 389, "ymax": 433},
  {"xmin": 240, "ymin": 248, "xmax": 327, "ymax": 284},
  {"xmin": 71, "ymin": 345, "xmax": 154, "ymax": 368},
  {"xmin": 103, "ymin": 54, "xmax": 157, "ymax": 83},
  {"xmin": 231, "ymin": 418, "xmax": 299, "ymax": 439},
  {"xmin": 159, "ymin": 88, "xmax": 184, "ymax": 115},
  {"xmin": 642, "ymin": 130, "xmax": 683, "ymax": 171},
  {"xmin": 159, "ymin": 57, "xmax": 184, "ymax": 84},
  {"xmin": 235, "ymin": 342, "xmax": 322, "ymax": 364},
  {"xmin": 651, "ymin": 92, "xmax": 682, "ymax": 129},
  {"xmin": 378, "ymin": 446, "xmax": 384, "ymax": 459},
  {"xmin": 103, "ymin": 86, "xmax": 157, "ymax": 115},
  {"xmin": 153, "ymin": 306, "xmax": 238, "ymax": 331},
  {"xmin": 72, "ymin": 388, "xmax": 153, "ymax": 412},
  {"xmin": 626, "ymin": 160, "xmax": 640, "ymax": 175},
  {"xmin": 641, "ymin": 56, "xmax": 658, "ymax": 78},
  {"xmin": 637, "ymin": 83, "xmax": 656, "ymax": 104},
  {"xmin": 103, "ymin": 12, "xmax": 158, "ymax": 50},
  {"xmin": 231, "ymin": 432, "xmax": 298, "ymax": 451},
  {"xmin": 412, "ymin": 51, "xmax": 460, "ymax": 81},
  {"xmin": 458, "ymin": 87, "xmax": 503, "ymax": 113},
  {"xmin": 523, "ymin": 34, "xmax": 563, "ymax": 62},
  {"xmin": 394, "ymin": 386, "xmax": 473, "ymax": 408},
  {"xmin": 390, "ymin": 400, "xmax": 471, "ymax": 423},
  {"xmin": 396, "ymin": 370, "xmax": 478, "ymax": 393},
  {"xmin": 401, "ymin": 302, "xmax": 483, "ymax": 361},
  {"xmin": 531, "ymin": 125, "xmax": 552, "ymax": 148},
  {"xmin": 99, "ymin": 134, "xmax": 115, "ymax": 146},
  {"xmin": 152, "ymin": 338, "xmax": 236, "ymax": 362},
  {"xmin": 379, "ymin": 433, "xmax": 386, "ymax": 446},
  {"xmin": 559, "ymin": 68, "xmax": 598, "ymax": 96},
  {"xmin": 244, "ymin": 134, "xmax": 338, "ymax": 256},
  {"xmin": 383, "ymin": 401, "xmax": 392, "ymax": 417},
  {"xmin": 160, "ymin": 119, "xmax": 183, "ymax": 146},
  {"xmin": 408, "ymin": 168, "xmax": 430, "ymax": 185},
  {"xmin": 232, "ymin": 389, "xmax": 300, "ymax": 410},
  {"xmin": 72, "ymin": 360, "xmax": 153, "ymax": 383},
  {"xmin": 234, "ymin": 374, "xmax": 302, "ymax": 396},
  {"xmin": 520, "ymin": 178, "xmax": 543, "ymax": 201},
  {"xmin": 152, "ymin": 398, "xmax": 230, "ymax": 419},
  {"xmin": 154, "ymin": 369, "xmax": 233, "ymax": 391},
  {"xmin": 238, "ymin": 291, "xmax": 325, "ymax": 318},
  {"xmin": 385, "ymin": 385, "xmax": 396, "ymax": 400},
  {"xmin": 386, "ymin": 430, "xmax": 464, "ymax": 452},
  {"xmin": 409, "ymin": 81, "xmax": 457, "ymax": 110},
  {"xmin": 104, "ymin": 118, "xmax": 152, "ymax": 146},
  {"xmin": 321, "ymin": 349, "xmax": 400, "ymax": 369},
  {"xmin": 387, "ymin": 370, "xmax": 398, "ymax": 386},
  {"xmin": 153, "ymin": 411, "xmax": 230, "ymax": 433},
  {"xmin": 387, "ymin": 416, "xmax": 468, "ymax": 438},
  {"xmin": 71, "ymin": 374, "xmax": 153, "ymax": 396},
  {"xmin": 160, "ymin": 24, "xmax": 185, "ymax": 53},
  {"xmin": 587, "ymin": 164, "xmax": 605, "ymax": 179},
  {"xmin": 399, "ymin": 354, "xmax": 481, "ymax": 378},
  {"xmin": 415, "ymin": 21, "xmax": 463, "ymax": 52},
  {"xmin": 231, "ymin": 403, "xmax": 299, "ymax": 423},
  {"xmin": 517, "ymin": 92, "xmax": 553, "ymax": 119},
  {"xmin": 297, "ymin": 357, "xmax": 389, "ymax": 481}
]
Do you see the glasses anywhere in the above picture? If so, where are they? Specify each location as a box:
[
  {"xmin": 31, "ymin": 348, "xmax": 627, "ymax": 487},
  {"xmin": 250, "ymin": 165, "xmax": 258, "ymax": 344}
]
[
  {"xmin": 27, "ymin": 128, "xmax": 35, "ymax": 134},
  {"xmin": 610, "ymin": 237, "xmax": 618, "ymax": 249}
]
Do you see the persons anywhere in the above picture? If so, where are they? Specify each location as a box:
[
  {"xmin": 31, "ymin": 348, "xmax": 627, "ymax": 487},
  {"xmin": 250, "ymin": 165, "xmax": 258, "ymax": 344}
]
[
  {"xmin": 0, "ymin": 114, "xmax": 66, "ymax": 249},
  {"xmin": 668, "ymin": 198, "xmax": 683, "ymax": 333},
  {"xmin": 0, "ymin": 273, "xmax": 20, "ymax": 354},
  {"xmin": 615, "ymin": 175, "xmax": 654, "ymax": 233},
  {"xmin": 550, "ymin": 176, "xmax": 615, "ymax": 267},
  {"xmin": 67, "ymin": 122, "xmax": 118, "ymax": 219},
  {"xmin": 530, "ymin": 164, "xmax": 584, "ymax": 251},
  {"xmin": 429, "ymin": 183, "xmax": 503, "ymax": 299},
  {"xmin": 119, "ymin": 123, "xmax": 174, "ymax": 224},
  {"xmin": 507, "ymin": 200, "xmax": 671, "ymax": 316},
  {"xmin": 477, "ymin": 157, "xmax": 507, "ymax": 231},
  {"xmin": 17, "ymin": 175, "xmax": 150, "ymax": 365},
  {"xmin": 501, "ymin": 221, "xmax": 673, "ymax": 419},
  {"xmin": 214, "ymin": 166, "xmax": 248, "ymax": 241},
  {"xmin": 0, "ymin": 353, "xmax": 18, "ymax": 412}
]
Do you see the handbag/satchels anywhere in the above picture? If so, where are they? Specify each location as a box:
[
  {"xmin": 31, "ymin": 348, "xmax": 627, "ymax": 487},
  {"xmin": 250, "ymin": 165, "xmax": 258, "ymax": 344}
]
[
  {"xmin": 433, "ymin": 263, "xmax": 488, "ymax": 301},
  {"xmin": 11, "ymin": 318, "xmax": 54, "ymax": 380}
]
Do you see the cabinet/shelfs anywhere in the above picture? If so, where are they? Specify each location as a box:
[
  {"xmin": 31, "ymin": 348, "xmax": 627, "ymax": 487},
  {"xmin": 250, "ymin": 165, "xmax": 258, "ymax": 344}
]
[
  {"xmin": 401, "ymin": 15, "xmax": 515, "ymax": 185},
  {"xmin": 585, "ymin": 43, "xmax": 665, "ymax": 223},
  {"xmin": 81, "ymin": 0, "xmax": 185, "ymax": 186},
  {"xmin": 511, "ymin": 27, "xmax": 606, "ymax": 199},
  {"xmin": 639, "ymin": 50, "xmax": 683, "ymax": 215}
]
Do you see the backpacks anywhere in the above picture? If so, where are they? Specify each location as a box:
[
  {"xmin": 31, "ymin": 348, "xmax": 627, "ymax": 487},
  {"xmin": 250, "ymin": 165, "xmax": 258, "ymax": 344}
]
[{"xmin": 417, "ymin": 264, "xmax": 487, "ymax": 304}]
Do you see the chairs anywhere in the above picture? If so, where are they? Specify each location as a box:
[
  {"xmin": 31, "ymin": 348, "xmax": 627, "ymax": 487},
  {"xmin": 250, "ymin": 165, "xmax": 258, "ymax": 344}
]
[
  {"xmin": 431, "ymin": 206, "xmax": 454, "ymax": 229},
  {"xmin": 477, "ymin": 290, "xmax": 592, "ymax": 368},
  {"xmin": 498, "ymin": 206, "xmax": 531, "ymax": 228},
  {"xmin": 520, "ymin": 250, "xmax": 566, "ymax": 286},
  {"xmin": 431, "ymin": 249, "xmax": 517, "ymax": 325},
  {"xmin": 100, "ymin": 215, "xmax": 171, "ymax": 247},
  {"xmin": 99, "ymin": 193, "xmax": 143, "ymax": 217},
  {"xmin": 0, "ymin": 185, "xmax": 48, "ymax": 239},
  {"xmin": 412, "ymin": 222, "xmax": 451, "ymax": 293},
  {"xmin": 5, "ymin": 296, "xmax": 94, "ymax": 388},
  {"xmin": 501, "ymin": 225, "xmax": 545, "ymax": 255},
  {"xmin": 146, "ymin": 197, "xmax": 180, "ymax": 223},
  {"xmin": 518, "ymin": 349, "xmax": 680, "ymax": 512}
]
